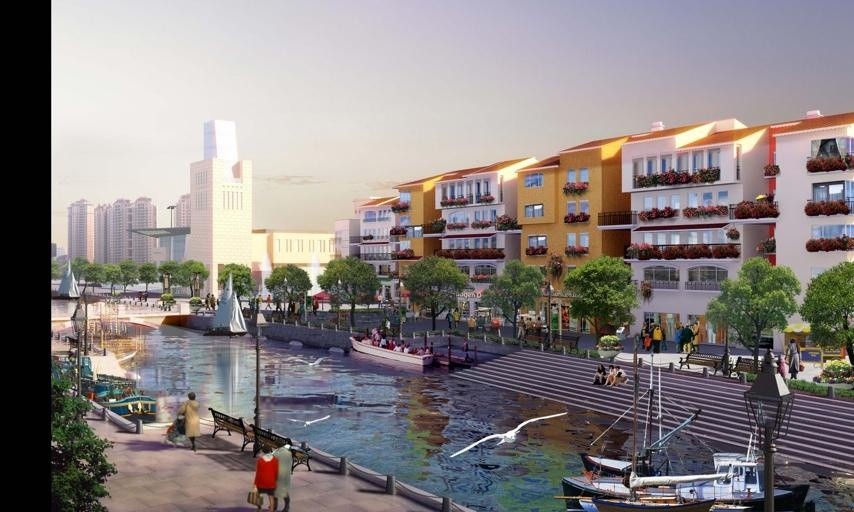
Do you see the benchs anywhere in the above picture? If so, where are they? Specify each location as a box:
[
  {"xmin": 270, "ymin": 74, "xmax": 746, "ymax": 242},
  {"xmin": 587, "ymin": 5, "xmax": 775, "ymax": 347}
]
[
  {"xmin": 518, "ymin": 329, "xmax": 580, "ymax": 355},
  {"xmin": 207, "ymin": 407, "xmax": 312, "ymax": 475},
  {"xmin": 679, "ymin": 350, "xmax": 765, "ymax": 379}
]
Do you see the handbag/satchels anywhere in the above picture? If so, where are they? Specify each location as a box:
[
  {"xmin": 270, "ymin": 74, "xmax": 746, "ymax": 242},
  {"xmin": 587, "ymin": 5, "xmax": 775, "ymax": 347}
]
[
  {"xmin": 247, "ymin": 491, "xmax": 263, "ymax": 506},
  {"xmin": 177, "ymin": 419, "xmax": 186, "ymax": 435}
]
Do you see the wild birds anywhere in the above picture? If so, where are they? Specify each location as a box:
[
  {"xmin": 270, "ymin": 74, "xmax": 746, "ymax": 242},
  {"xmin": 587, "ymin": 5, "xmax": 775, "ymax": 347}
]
[
  {"xmin": 293, "ymin": 356, "xmax": 333, "ymax": 368},
  {"xmin": 285, "ymin": 414, "xmax": 331, "ymax": 428},
  {"xmin": 449, "ymin": 411, "xmax": 568, "ymax": 458}
]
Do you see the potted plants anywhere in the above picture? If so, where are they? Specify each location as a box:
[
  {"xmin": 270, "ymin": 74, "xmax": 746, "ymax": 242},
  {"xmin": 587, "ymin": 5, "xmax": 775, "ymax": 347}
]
[
  {"xmin": 594, "ymin": 335, "xmax": 625, "ymax": 363},
  {"xmin": 813, "ymin": 360, "xmax": 854, "ymax": 394},
  {"xmin": 189, "ymin": 296, "xmax": 204, "ymax": 312}
]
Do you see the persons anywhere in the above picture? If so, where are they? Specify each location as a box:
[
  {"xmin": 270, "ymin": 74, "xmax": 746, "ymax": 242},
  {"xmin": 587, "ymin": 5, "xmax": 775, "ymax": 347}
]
[
  {"xmin": 445, "ymin": 308, "xmax": 550, "ymax": 343},
  {"xmin": 361, "ymin": 326, "xmax": 429, "ymax": 361},
  {"xmin": 253, "ymin": 445, "xmax": 277, "ymax": 512},
  {"xmin": 177, "ymin": 390, "xmax": 203, "ymax": 452},
  {"xmin": 776, "ymin": 353, "xmax": 788, "ymax": 379},
  {"xmin": 785, "ymin": 338, "xmax": 799, "ymax": 379},
  {"xmin": 604, "ymin": 365, "xmax": 616, "ymax": 387},
  {"xmin": 764, "ymin": 348, "xmax": 775, "ymax": 364},
  {"xmin": 611, "ymin": 366, "xmax": 627, "ymax": 390},
  {"xmin": 591, "ymin": 363, "xmax": 605, "ymax": 384},
  {"xmin": 271, "ymin": 439, "xmax": 292, "ymax": 511},
  {"xmin": 641, "ymin": 320, "xmax": 701, "ymax": 354}
]
